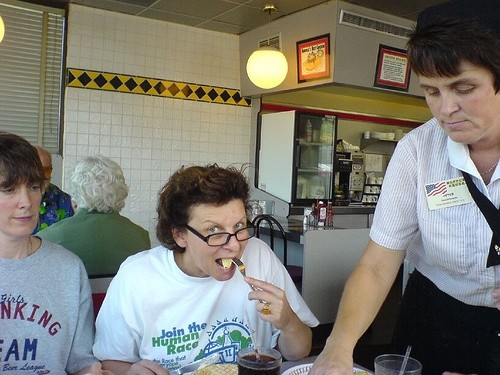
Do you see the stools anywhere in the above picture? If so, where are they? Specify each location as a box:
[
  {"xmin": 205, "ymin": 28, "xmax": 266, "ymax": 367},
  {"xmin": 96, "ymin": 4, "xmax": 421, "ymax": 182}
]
[{"xmin": 253, "ymin": 214, "xmax": 303, "ymax": 282}]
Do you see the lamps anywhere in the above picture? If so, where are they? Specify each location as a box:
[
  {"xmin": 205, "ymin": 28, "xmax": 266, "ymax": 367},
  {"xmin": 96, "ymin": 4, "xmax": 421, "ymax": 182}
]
[{"xmin": 247, "ymin": 4, "xmax": 288, "ymax": 90}]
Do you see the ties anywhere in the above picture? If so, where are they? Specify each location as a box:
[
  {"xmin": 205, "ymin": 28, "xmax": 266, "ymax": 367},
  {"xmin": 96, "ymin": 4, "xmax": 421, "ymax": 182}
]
[{"xmin": 460, "ymin": 171, "xmax": 500, "ymax": 268}]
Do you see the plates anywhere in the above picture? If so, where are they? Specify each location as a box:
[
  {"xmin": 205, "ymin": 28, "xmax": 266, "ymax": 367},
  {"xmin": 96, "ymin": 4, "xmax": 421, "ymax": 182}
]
[{"xmin": 281, "ymin": 363, "xmax": 372, "ymax": 375}]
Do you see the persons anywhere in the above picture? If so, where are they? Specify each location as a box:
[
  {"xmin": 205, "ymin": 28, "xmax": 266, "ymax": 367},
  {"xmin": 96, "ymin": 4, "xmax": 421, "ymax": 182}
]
[
  {"xmin": 310, "ymin": 0, "xmax": 500, "ymax": 375},
  {"xmin": 0, "ymin": 130, "xmax": 111, "ymax": 375},
  {"xmin": 32, "ymin": 145, "xmax": 79, "ymax": 237},
  {"xmin": 35, "ymin": 155, "xmax": 151, "ymax": 277},
  {"xmin": 91, "ymin": 162, "xmax": 320, "ymax": 375}
]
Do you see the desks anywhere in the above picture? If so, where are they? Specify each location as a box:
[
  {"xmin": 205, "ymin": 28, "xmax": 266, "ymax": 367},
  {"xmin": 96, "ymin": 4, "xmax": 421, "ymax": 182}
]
[{"xmin": 260, "ymin": 221, "xmax": 347, "ymax": 245}]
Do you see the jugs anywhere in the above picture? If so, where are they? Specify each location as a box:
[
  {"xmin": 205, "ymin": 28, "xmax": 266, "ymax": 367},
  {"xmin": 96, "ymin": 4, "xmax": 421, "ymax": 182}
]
[{"xmin": 247, "ymin": 199, "xmax": 276, "ymax": 224}]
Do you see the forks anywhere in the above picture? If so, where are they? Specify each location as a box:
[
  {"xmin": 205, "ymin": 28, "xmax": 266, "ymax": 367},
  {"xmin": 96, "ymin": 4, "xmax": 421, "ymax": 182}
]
[{"xmin": 231, "ymin": 258, "xmax": 265, "ymax": 303}]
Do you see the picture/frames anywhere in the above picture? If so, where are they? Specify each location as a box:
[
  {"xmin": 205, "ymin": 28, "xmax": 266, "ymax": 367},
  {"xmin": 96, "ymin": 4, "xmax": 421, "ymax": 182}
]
[{"xmin": 296, "ymin": 32, "xmax": 331, "ymax": 83}]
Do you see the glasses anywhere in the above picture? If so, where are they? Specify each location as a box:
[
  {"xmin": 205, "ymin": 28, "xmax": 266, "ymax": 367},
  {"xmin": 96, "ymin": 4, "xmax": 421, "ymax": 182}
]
[
  {"xmin": 42, "ymin": 167, "xmax": 53, "ymax": 180},
  {"xmin": 184, "ymin": 219, "xmax": 258, "ymax": 245}
]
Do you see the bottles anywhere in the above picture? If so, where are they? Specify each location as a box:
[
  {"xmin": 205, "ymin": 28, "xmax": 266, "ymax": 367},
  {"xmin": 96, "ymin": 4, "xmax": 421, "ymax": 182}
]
[
  {"xmin": 302, "ymin": 147, "xmax": 332, "ymax": 168},
  {"xmin": 320, "ymin": 118, "xmax": 333, "ymax": 144},
  {"xmin": 303, "ymin": 216, "xmax": 308, "ymax": 224},
  {"xmin": 318, "ymin": 201, "xmax": 327, "ymax": 222},
  {"xmin": 310, "ymin": 207, "xmax": 318, "ymax": 227},
  {"xmin": 296, "ymin": 174, "xmax": 324, "ymax": 199},
  {"xmin": 304, "ymin": 118, "xmax": 312, "ymax": 143},
  {"xmin": 327, "ymin": 202, "xmax": 333, "ymax": 228}
]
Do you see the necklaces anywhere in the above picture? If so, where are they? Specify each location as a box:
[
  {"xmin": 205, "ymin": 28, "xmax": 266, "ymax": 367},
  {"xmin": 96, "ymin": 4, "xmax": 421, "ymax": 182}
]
[
  {"xmin": 479, "ymin": 162, "xmax": 497, "ymax": 174},
  {"xmin": 29, "ymin": 236, "xmax": 32, "ymax": 255}
]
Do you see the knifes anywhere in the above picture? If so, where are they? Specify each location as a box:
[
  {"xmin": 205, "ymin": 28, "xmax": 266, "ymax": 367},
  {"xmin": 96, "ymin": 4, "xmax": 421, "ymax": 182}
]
[{"xmin": 169, "ymin": 353, "xmax": 219, "ymax": 375}]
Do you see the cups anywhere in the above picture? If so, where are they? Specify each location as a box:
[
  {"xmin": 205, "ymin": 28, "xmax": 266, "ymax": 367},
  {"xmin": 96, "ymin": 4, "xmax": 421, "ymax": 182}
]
[
  {"xmin": 372, "ymin": 186, "xmax": 380, "ymax": 193},
  {"xmin": 373, "ymin": 196, "xmax": 378, "ymax": 202},
  {"xmin": 237, "ymin": 346, "xmax": 282, "ymax": 375},
  {"xmin": 367, "ymin": 196, "xmax": 373, "ymax": 202},
  {"xmin": 364, "ymin": 186, "xmax": 373, "ymax": 193},
  {"xmin": 374, "ymin": 354, "xmax": 421, "ymax": 374},
  {"xmin": 377, "ymin": 177, "xmax": 383, "ymax": 184},
  {"xmin": 376, "ymin": 131, "xmax": 409, "ymax": 139},
  {"xmin": 368, "ymin": 176, "xmax": 377, "ymax": 184},
  {"xmin": 363, "ymin": 195, "xmax": 367, "ymax": 202},
  {"xmin": 364, "ymin": 132, "xmax": 370, "ymax": 140}
]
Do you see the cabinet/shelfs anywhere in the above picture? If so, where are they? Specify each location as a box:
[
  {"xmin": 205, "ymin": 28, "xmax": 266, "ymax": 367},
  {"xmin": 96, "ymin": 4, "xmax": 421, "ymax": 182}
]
[{"xmin": 261, "ymin": 110, "xmax": 338, "ymax": 209}]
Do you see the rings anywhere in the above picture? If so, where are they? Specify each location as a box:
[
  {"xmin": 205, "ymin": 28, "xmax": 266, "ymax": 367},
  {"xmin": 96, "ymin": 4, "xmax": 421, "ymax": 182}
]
[{"xmin": 262, "ymin": 304, "xmax": 271, "ymax": 314}]
